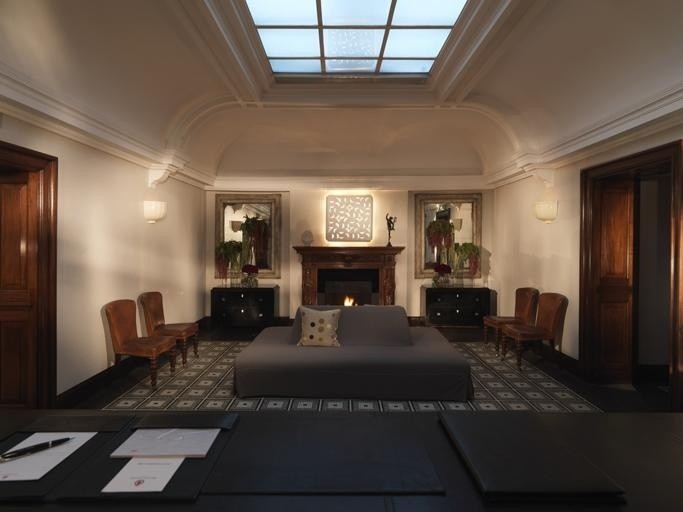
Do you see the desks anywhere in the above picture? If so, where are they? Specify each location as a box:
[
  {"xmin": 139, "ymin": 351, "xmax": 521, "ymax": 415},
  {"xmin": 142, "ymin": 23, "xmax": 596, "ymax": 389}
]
[{"xmin": 0, "ymin": 412, "xmax": 683, "ymax": 512}]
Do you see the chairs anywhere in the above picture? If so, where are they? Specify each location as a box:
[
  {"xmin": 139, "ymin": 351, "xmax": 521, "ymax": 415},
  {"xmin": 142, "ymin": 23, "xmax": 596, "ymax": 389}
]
[
  {"xmin": 501, "ymin": 292, "xmax": 568, "ymax": 372},
  {"xmin": 138, "ymin": 290, "xmax": 201, "ymax": 368},
  {"xmin": 104, "ymin": 299, "xmax": 178, "ymax": 387},
  {"xmin": 482, "ymin": 287, "xmax": 539, "ymax": 354}
]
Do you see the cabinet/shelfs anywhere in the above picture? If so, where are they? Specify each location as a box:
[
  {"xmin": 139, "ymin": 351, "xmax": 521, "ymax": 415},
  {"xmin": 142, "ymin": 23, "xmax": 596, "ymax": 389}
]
[
  {"xmin": 419, "ymin": 284, "xmax": 496, "ymax": 327},
  {"xmin": 211, "ymin": 284, "xmax": 279, "ymax": 335}
]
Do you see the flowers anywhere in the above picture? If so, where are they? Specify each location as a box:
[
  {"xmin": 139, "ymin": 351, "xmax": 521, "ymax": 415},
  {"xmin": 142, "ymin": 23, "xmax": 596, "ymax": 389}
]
[
  {"xmin": 454, "ymin": 242, "xmax": 480, "ymax": 276},
  {"xmin": 241, "ymin": 263, "xmax": 258, "ymax": 276},
  {"xmin": 432, "ymin": 263, "xmax": 451, "ymax": 274},
  {"xmin": 426, "ymin": 218, "xmax": 455, "ymax": 252}
]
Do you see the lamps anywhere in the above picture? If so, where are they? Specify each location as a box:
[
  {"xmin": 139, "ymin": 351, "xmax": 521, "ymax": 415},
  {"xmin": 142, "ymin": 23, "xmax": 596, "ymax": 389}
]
[
  {"xmin": 142, "ymin": 199, "xmax": 168, "ymax": 224},
  {"xmin": 531, "ymin": 199, "xmax": 559, "ymax": 224}
]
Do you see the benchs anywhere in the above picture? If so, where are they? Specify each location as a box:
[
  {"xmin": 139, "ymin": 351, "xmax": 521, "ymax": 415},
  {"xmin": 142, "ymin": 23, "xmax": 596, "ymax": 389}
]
[{"xmin": 232, "ymin": 305, "xmax": 475, "ymax": 403}]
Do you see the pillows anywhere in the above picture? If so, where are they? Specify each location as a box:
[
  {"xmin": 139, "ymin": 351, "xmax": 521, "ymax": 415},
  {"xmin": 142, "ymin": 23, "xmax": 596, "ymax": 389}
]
[{"xmin": 297, "ymin": 305, "xmax": 342, "ymax": 348}]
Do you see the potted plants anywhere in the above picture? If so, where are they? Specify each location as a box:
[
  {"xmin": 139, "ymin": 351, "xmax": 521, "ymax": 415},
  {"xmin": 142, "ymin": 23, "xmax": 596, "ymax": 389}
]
[
  {"xmin": 213, "ymin": 238, "xmax": 242, "ymax": 288},
  {"xmin": 239, "ymin": 213, "xmax": 263, "ymax": 266}
]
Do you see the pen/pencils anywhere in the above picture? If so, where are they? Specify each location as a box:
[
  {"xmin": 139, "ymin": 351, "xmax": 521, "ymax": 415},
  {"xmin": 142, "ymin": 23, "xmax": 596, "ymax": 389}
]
[{"xmin": 0, "ymin": 436, "xmax": 75, "ymax": 463}]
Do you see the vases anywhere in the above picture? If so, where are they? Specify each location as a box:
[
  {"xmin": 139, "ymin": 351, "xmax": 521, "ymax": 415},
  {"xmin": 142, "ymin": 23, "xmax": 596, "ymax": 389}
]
[
  {"xmin": 451, "ymin": 268, "xmax": 474, "ymax": 287},
  {"xmin": 240, "ymin": 273, "xmax": 258, "ymax": 288},
  {"xmin": 435, "ymin": 238, "xmax": 448, "ymax": 266},
  {"xmin": 432, "ymin": 274, "xmax": 451, "ymax": 289}
]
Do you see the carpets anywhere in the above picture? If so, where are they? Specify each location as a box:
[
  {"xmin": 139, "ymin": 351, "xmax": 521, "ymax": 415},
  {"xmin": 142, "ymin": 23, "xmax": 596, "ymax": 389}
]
[{"xmin": 101, "ymin": 339, "xmax": 604, "ymax": 415}]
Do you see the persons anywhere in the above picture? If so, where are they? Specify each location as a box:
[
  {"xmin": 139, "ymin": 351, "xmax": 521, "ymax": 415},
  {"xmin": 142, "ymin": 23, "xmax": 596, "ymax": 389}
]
[{"xmin": 386, "ymin": 213, "xmax": 397, "ymax": 241}]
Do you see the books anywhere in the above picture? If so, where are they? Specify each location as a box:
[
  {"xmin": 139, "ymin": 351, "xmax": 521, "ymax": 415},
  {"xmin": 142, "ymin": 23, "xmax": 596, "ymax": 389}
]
[
  {"xmin": 109, "ymin": 428, "xmax": 222, "ymax": 458},
  {"xmin": 102, "ymin": 455, "xmax": 186, "ymax": 493},
  {"xmin": 0, "ymin": 432, "xmax": 98, "ymax": 482}
]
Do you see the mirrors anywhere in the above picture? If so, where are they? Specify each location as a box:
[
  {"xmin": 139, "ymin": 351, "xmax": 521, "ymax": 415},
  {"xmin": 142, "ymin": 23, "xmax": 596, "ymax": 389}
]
[
  {"xmin": 414, "ymin": 193, "xmax": 482, "ymax": 278},
  {"xmin": 215, "ymin": 193, "xmax": 282, "ymax": 279}
]
